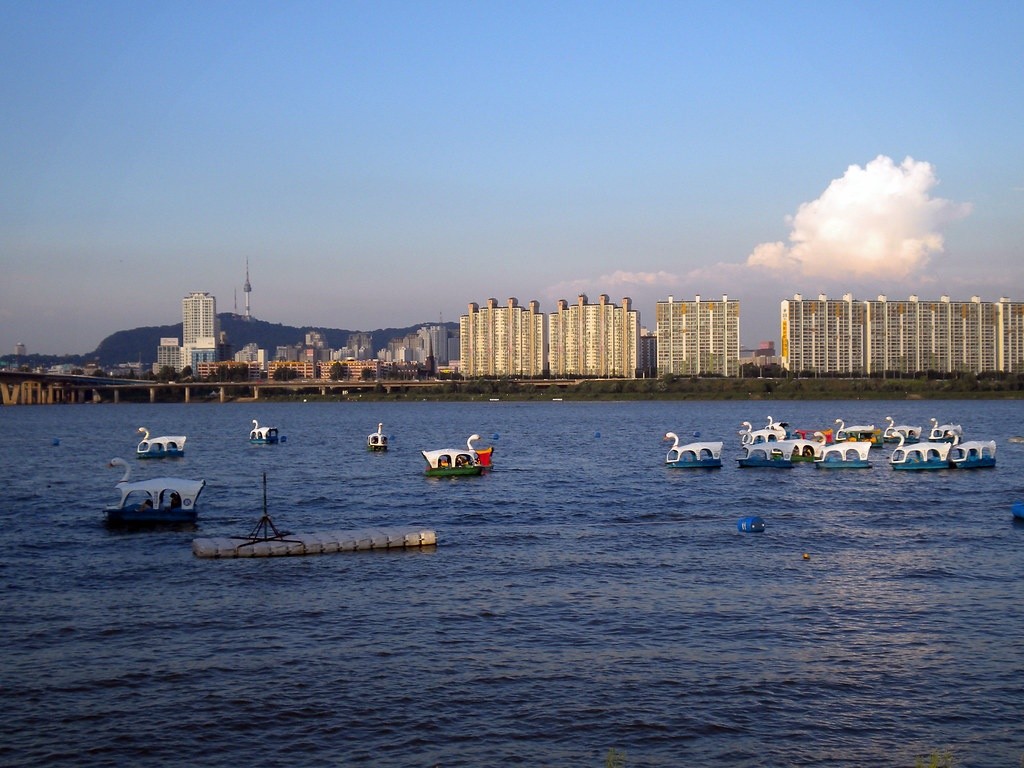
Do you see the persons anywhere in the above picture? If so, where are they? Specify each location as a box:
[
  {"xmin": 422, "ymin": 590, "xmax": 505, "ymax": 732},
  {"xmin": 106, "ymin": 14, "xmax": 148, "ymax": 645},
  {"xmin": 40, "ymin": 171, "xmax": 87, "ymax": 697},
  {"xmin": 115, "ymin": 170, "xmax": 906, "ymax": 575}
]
[
  {"xmin": 438, "ymin": 457, "xmax": 480, "ymax": 468},
  {"xmin": 170, "ymin": 493, "xmax": 181, "ymax": 509},
  {"xmin": 372, "ymin": 437, "xmax": 388, "ymax": 444},
  {"xmin": 849, "ymin": 432, "xmax": 854, "ymax": 438}
]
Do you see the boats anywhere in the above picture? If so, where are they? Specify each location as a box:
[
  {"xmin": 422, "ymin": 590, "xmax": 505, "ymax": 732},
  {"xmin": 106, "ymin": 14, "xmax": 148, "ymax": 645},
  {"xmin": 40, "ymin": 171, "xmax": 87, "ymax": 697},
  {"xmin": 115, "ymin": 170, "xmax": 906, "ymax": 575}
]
[
  {"xmin": 812, "ymin": 430, "xmax": 874, "ymax": 469},
  {"xmin": 367, "ymin": 423, "xmax": 389, "ymax": 451},
  {"xmin": 771, "ymin": 434, "xmax": 821, "ymax": 462},
  {"xmin": 792, "ymin": 429, "xmax": 835, "ymax": 445},
  {"xmin": 135, "ymin": 427, "xmax": 186, "ymax": 461},
  {"xmin": 421, "ymin": 434, "xmax": 495, "ymax": 474},
  {"xmin": 930, "ymin": 417, "xmax": 962, "ymax": 442},
  {"xmin": 250, "ymin": 420, "xmax": 278, "ymax": 444},
  {"xmin": 733, "ymin": 430, "xmax": 796, "ymax": 468},
  {"xmin": 740, "ymin": 415, "xmax": 791, "ymax": 447},
  {"xmin": 946, "ymin": 431, "xmax": 996, "ymax": 469},
  {"xmin": 663, "ymin": 432, "xmax": 723, "ymax": 469},
  {"xmin": 889, "ymin": 432, "xmax": 949, "ymax": 470},
  {"xmin": 885, "ymin": 416, "xmax": 922, "ymax": 442},
  {"xmin": 832, "ymin": 418, "xmax": 884, "ymax": 448},
  {"xmin": 104, "ymin": 456, "xmax": 206, "ymax": 531}
]
